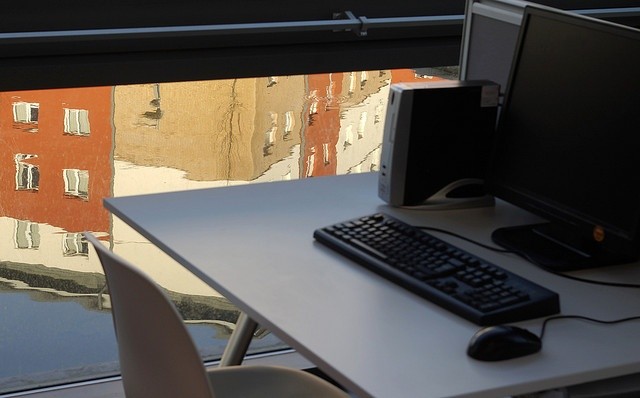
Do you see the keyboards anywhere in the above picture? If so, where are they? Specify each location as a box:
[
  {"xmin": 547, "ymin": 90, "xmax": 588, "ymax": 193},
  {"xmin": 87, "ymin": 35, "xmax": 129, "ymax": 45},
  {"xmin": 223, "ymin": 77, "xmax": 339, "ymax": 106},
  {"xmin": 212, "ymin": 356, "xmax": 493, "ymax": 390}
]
[{"xmin": 314, "ymin": 213, "xmax": 562, "ymax": 327}]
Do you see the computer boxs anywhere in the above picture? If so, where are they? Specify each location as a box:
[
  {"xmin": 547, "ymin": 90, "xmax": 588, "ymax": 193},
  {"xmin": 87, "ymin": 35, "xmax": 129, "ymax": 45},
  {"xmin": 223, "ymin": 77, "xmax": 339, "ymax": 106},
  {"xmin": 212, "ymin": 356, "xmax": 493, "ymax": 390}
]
[{"xmin": 378, "ymin": 79, "xmax": 501, "ymax": 210}]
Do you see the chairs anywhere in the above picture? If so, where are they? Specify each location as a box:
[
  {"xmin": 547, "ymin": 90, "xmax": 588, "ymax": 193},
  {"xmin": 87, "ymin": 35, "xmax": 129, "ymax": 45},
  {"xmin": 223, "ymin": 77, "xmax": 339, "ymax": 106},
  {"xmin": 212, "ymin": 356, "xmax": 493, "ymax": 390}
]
[{"xmin": 84, "ymin": 233, "xmax": 349, "ymax": 396}]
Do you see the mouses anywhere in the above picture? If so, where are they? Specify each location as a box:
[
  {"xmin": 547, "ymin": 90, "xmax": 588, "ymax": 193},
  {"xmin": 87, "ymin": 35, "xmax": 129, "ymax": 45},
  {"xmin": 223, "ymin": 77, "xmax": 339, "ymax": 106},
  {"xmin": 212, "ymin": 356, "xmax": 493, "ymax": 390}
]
[{"xmin": 467, "ymin": 323, "xmax": 541, "ymax": 362}]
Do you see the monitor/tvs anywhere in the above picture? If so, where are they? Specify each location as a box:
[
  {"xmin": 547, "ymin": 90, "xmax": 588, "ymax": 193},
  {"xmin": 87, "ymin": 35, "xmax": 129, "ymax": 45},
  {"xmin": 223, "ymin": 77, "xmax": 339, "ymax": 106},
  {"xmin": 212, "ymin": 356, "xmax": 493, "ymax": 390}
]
[{"xmin": 493, "ymin": 5, "xmax": 640, "ymax": 276}]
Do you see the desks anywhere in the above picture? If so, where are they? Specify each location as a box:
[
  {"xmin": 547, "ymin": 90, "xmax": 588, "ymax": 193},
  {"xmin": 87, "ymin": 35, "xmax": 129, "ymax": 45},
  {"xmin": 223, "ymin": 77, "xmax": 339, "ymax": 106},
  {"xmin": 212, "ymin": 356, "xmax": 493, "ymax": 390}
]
[{"xmin": 103, "ymin": 169, "xmax": 640, "ymax": 398}]
